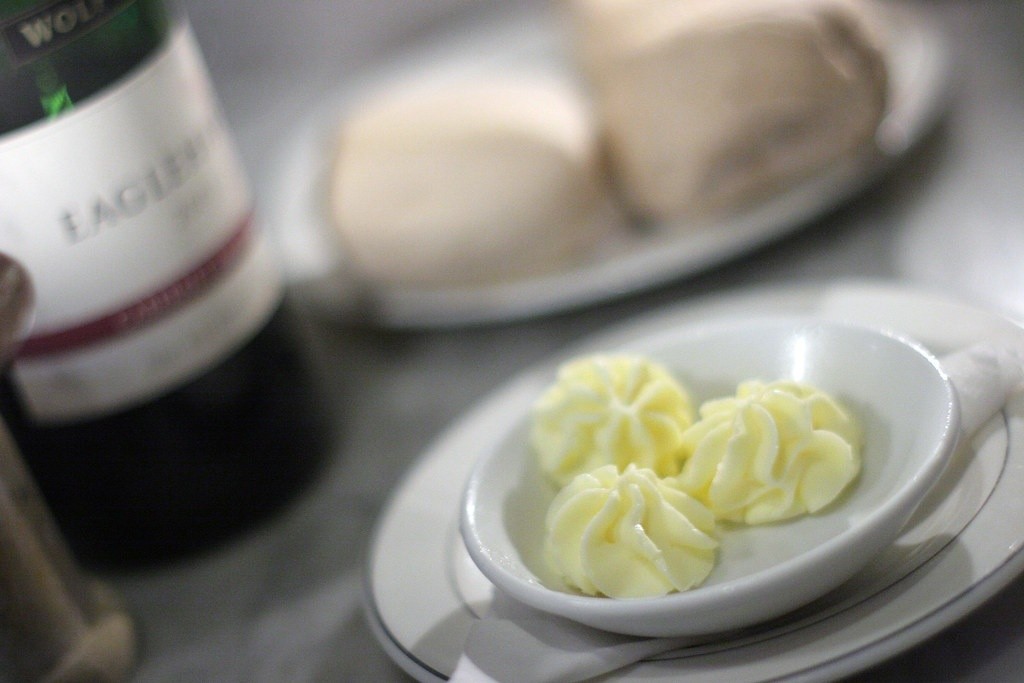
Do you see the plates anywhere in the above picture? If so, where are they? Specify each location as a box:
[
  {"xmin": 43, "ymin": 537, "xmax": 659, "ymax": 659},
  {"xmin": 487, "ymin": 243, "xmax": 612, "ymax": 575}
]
[
  {"xmin": 361, "ymin": 282, "xmax": 1024, "ymax": 683},
  {"xmin": 269, "ymin": 0, "xmax": 952, "ymax": 332}
]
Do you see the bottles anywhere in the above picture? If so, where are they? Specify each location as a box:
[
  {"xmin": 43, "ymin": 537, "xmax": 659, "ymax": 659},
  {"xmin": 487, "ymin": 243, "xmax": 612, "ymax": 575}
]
[{"xmin": 0, "ymin": 0, "xmax": 337, "ymax": 578}]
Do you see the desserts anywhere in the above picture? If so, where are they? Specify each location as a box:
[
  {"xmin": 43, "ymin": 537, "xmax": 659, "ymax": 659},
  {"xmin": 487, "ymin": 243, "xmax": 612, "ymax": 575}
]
[{"xmin": 527, "ymin": 353, "xmax": 863, "ymax": 600}]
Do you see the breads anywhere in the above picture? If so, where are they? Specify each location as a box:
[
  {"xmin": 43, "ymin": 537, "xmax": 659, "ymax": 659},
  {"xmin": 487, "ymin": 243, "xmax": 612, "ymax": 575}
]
[{"xmin": 321, "ymin": 0, "xmax": 894, "ymax": 290}]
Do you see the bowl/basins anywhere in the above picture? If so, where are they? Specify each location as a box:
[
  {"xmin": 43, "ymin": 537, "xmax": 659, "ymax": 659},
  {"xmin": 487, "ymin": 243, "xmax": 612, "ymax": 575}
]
[{"xmin": 461, "ymin": 311, "xmax": 964, "ymax": 642}]
